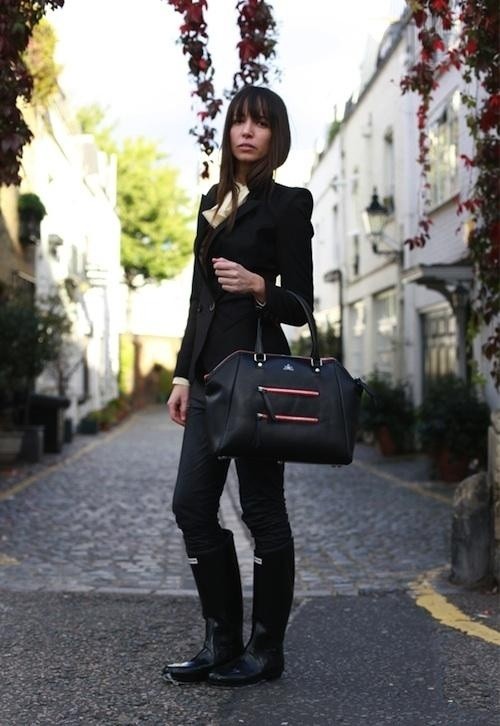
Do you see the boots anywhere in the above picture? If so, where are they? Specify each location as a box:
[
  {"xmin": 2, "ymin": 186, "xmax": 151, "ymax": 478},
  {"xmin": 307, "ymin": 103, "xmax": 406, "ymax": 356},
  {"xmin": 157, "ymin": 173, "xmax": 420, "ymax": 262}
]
[
  {"xmin": 206, "ymin": 535, "xmax": 294, "ymax": 688},
  {"xmin": 164, "ymin": 527, "xmax": 244, "ymax": 683}
]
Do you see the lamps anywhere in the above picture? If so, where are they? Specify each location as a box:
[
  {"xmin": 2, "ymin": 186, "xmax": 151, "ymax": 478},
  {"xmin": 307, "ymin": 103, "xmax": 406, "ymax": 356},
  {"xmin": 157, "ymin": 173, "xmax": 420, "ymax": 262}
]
[{"xmin": 361, "ymin": 184, "xmax": 404, "ymax": 263}]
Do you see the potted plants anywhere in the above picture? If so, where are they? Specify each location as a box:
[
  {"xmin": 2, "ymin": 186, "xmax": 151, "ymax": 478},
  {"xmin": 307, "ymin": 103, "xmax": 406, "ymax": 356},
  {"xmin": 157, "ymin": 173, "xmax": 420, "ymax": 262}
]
[
  {"xmin": 18, "ymin": 191, "xmax": 45, "ymax": 247},
  {"xmin": 0, "ymin": 289, "xmax": 175, "ymax": 461},
  {"xmin": 353, "ymin": 369, "xmax": 489, "ymax": 485}
]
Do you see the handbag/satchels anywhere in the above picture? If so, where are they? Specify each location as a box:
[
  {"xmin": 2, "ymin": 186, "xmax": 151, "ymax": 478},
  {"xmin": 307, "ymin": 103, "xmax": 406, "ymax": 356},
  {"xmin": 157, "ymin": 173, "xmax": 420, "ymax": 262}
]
[{"xmin": 202, "ymin": 287, "xmax": 373, "ymax": 466}]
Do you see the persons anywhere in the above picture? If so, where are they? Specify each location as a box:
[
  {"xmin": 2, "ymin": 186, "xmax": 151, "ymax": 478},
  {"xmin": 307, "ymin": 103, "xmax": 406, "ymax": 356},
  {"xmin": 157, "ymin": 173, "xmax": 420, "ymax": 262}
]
[{"xmin": 159, "ymin": 83, "xmax": 315, "ymax": 692}]
